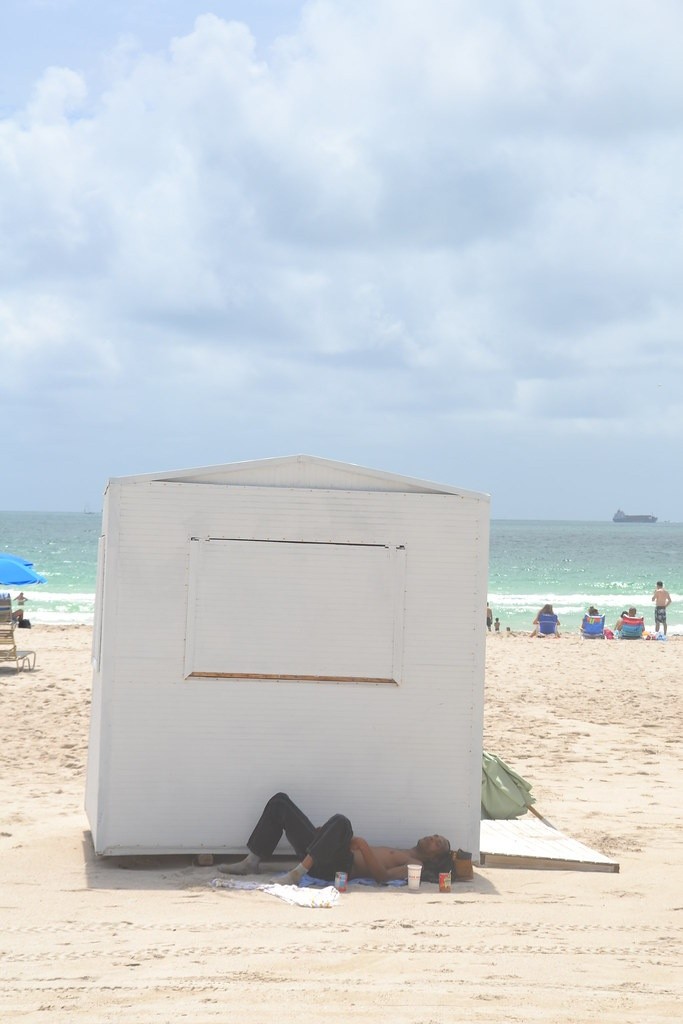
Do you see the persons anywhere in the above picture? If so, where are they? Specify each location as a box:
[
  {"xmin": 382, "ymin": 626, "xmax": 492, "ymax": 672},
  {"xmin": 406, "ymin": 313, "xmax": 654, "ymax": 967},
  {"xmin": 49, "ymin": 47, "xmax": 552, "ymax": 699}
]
[
  {"xmin": 12, "ymin": 608, "xmax": 23, "ymax": 624},
  {"xmin": 494, "ymin": 618, "xmax": 500, "ymax": 631},
  {"xmin": 13, "ymin": 592, "xmax": 28, "ymax": 605},
  {"xmin": 530, "ymin": 604, "xmax": 561, "ymax": 638},
  {"xmin": 218, "ymin": 791, "xmax": 451, "ymax": 887},
  {"xmin": 652, "ymin": 581, "xmax": 672, "ymax": 635},
  {"xmin": 617, "ymin": 607, "xmax": 645, "ymax": 631},
  {"xmin": 487, "ymin": 602, "xmax": 493, "ymax": 631},
  {"xmin": 582, "ymin": 606, "xmax": 599, "ymax": 628},
  {"xmin": 614, "ymin": 610, "xmax": 628, "ymax": 630}
]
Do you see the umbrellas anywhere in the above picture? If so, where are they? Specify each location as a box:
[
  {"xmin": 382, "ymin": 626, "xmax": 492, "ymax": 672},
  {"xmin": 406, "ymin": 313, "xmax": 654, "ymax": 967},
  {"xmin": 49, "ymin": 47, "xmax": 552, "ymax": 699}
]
[{"xmin": 0, "ymin": 551, "xmax": 48, "ymax": 587}]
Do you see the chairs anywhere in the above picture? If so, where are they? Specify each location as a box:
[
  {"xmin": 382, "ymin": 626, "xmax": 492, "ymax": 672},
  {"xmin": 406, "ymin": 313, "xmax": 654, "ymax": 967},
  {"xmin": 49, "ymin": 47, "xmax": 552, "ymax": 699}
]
[
  {"xmin": 536, "ymin": 612, "xmax": 559, "ymax": 639},
  {"xmin": 0, "ymin": 629, "xmax": 36, "ymax": 675},
  {"xmin": 617, "ymin": 614, "xmax": 645, "ymax": 640},
  {"xmin": 580, "ymin": 613, "xmax": 606, "ymax": 640},
  {"xmin": 0, "ymin": 598, "xmax": 17, "ymax": 630}
]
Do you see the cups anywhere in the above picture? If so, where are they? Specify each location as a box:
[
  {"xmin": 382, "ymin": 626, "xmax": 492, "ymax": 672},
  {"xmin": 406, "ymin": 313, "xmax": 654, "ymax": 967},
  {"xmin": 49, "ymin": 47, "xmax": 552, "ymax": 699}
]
[
  {"xmin": 439, "ymin": 873, "xmax": 451, "ymax": 893},
  {"xmin": 334, "ymin": 872, "xmax": 348, "ymax": 892},
  {"xmin": 407, "ymin": 864, "xmax": 422, "ymax": 891}
]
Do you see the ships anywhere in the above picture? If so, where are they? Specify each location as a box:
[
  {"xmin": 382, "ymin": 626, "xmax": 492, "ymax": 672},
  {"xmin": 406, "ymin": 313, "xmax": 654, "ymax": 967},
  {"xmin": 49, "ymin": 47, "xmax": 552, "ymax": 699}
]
[{"xmin": 612, "ymin": 508, "xmax": 658, "ymax": 524}]
